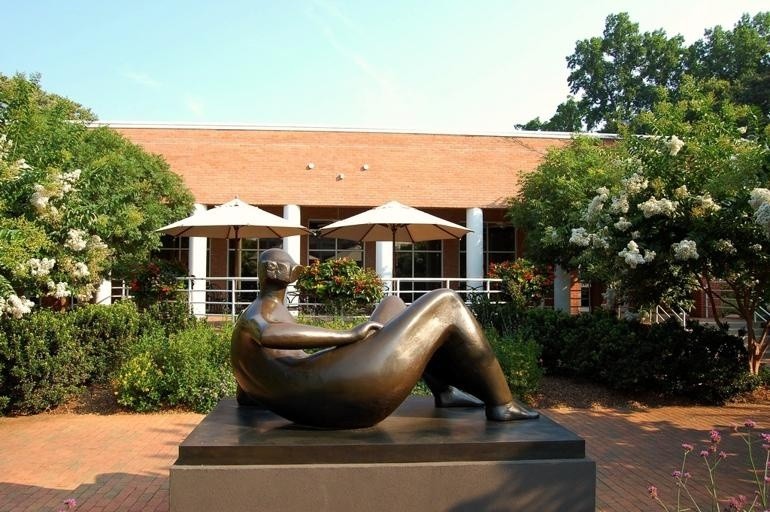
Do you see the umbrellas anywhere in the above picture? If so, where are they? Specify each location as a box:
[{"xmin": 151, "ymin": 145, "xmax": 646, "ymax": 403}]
[
  {"xmin": 152, "ymin": 195, "xmax": 317, "ymax": 301},
  {"xmin": 318, "ymin": 200, "xmax": 474, "ymax": 295}
]
